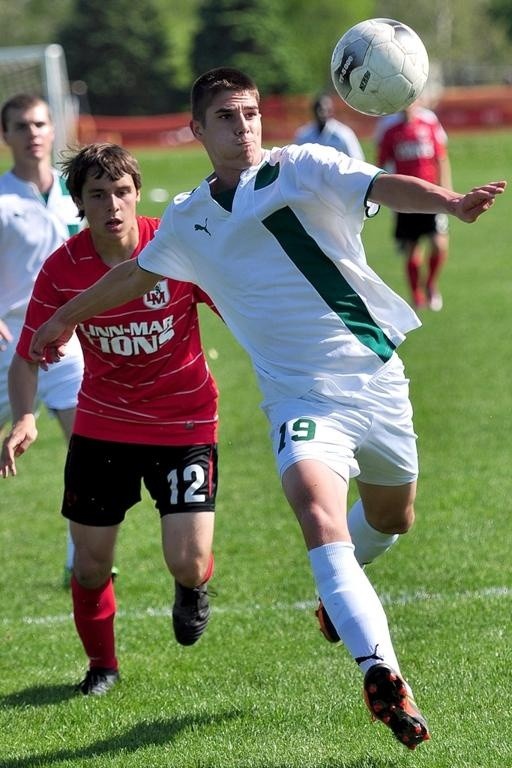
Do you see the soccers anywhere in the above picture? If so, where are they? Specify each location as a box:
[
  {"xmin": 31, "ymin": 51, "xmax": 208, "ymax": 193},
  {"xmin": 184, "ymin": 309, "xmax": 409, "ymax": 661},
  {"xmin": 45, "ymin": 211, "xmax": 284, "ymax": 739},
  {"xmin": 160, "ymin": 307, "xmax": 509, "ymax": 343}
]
[{"xmin": 330, "ymin": 17, "xmax": 430, "ymax": 116}]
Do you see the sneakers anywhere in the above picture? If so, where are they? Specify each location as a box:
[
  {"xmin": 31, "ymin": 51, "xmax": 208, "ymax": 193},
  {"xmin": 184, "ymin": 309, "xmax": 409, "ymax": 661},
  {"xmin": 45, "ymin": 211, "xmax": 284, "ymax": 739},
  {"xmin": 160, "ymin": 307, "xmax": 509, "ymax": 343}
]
[
  {"xmin": 74, "ymin": 667, "xmax": 122, "ymax": 699},
  {"xmin": 411, "ymin": 293, "xmax": 443, "ymax": 312},
  {"xmin": 363, "ymin": 663, "xmax": 432, "ymax": 751},
  {"xmin": 172, "ymin": 575, "xmax": 211, "ymax": 646},
  {"xmin": 314, "ymin": 596, "xmax": 341, "ymax": 643}
]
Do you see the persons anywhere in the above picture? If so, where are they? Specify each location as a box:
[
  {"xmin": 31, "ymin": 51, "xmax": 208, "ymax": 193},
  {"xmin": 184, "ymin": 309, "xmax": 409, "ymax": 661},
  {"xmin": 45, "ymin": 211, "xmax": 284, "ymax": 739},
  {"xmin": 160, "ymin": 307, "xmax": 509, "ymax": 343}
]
[
  {"xmin": 295, "ymin": 94, "xmax": 367, "ymax": 162},
  {"xmin": 374, "ymin": 101, "xmax": 452, "ymax": 310},
  {"xmin": 0, "ymin": 94, "xmax": 122, "ymax": 578},
  {"xmin": 0, "ymin": 140, "xmax": 226, "ymax": 696},
  {"xmin": 24, "ymin": 67, "xmax": 507, "ymax": 749}
]
